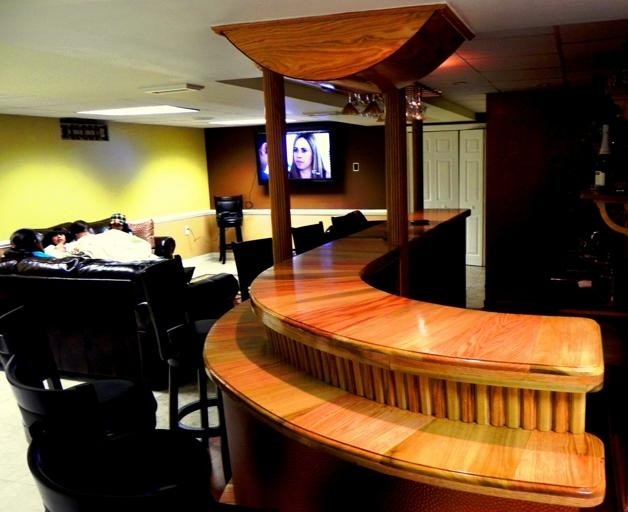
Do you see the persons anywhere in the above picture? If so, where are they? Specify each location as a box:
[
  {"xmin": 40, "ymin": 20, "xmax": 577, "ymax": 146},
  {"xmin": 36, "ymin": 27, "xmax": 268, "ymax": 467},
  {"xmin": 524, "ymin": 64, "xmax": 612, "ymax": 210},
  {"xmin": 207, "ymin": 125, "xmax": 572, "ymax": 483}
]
[
  {"xmin": 8, "ymin": 228, "xmax": 55, "ymax": 259},
  {"xmin": 101, "ymin": 212, "xmax": 133, "ymax": 237},
  {"xmin": 42, "ymin": 224, "xmax": 75, "ymax": 249},
  {"xmin": 288, "ymin": 133, "xmax": 331, "ymax": 179},
  {"xmin": 258, "ymin": 133, "xmax": 269, "ymax": 181},
  {"xmin": 71, "ymin": 220, "xmax": 90, "ymax": 238}
]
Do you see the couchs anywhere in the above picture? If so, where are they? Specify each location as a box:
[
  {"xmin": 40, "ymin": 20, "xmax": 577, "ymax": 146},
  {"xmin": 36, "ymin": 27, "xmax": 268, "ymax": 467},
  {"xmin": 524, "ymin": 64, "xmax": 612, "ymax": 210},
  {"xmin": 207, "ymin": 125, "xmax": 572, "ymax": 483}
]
[{"xmin": 0, "ymin": 217, "xmax": 240, "ymax": 385}]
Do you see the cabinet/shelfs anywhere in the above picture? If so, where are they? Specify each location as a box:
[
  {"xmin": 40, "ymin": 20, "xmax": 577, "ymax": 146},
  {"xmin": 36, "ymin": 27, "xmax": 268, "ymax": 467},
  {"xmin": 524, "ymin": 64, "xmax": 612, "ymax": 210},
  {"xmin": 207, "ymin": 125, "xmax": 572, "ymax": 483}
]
[{"xmin": 594, "ymin": 123, "xmax": 614, "ymax": 193}]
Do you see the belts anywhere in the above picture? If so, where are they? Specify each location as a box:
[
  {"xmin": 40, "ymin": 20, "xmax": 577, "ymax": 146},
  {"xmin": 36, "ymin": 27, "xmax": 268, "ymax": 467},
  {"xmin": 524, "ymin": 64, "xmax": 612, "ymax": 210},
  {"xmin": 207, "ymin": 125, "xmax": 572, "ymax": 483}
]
[{"xmin": 348, "ymin": 95, "xmax": 426, "ymax": 121}]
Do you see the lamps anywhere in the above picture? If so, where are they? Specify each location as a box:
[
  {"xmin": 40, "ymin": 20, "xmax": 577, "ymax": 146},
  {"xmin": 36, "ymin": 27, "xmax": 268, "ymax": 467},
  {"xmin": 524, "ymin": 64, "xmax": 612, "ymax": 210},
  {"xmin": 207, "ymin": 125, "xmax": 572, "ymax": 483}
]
[{"xmin": 4, "ymin": 353, "xmax": 213, "ymax": 511}]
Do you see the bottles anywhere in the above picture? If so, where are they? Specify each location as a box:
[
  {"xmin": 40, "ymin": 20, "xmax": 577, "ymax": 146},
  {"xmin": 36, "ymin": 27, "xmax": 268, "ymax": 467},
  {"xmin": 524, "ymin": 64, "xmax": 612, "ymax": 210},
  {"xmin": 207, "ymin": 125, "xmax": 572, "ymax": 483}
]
[{"xmin": 109, "ymin": 213, "xmax": 127, "ymax": 226}]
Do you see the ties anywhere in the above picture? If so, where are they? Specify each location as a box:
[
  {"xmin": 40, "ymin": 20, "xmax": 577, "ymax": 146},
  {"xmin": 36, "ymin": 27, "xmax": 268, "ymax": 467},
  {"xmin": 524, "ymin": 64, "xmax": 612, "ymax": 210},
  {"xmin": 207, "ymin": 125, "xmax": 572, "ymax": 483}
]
[{"xmin": 253, "ymin": 128, "xmax": 337, "ymax": 186}]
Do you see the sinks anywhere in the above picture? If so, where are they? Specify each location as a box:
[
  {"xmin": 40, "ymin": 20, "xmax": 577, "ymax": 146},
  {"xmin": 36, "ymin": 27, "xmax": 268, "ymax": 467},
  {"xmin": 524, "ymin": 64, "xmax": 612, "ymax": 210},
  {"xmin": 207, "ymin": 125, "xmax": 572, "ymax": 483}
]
[{"xmin": 0, "ymin": 217, "xmax": 240, "ymax": 385}]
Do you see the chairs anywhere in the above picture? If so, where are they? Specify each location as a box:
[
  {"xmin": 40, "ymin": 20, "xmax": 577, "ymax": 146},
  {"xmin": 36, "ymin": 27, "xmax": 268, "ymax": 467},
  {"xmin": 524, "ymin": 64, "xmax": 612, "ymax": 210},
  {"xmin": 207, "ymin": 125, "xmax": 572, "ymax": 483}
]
[
  {"xmin": 132, "ymin": 255, "xmax": 232, "ymax": 482},
  {"xmin": 4, "ymin": 353, "xmax": 213, "ymax": 511},
  {"xmin": 230, "ymin": 211, "xmax": 370, "ymax": 299},
  {"xmin": 214, "ymin": 195, "xmax": 244, "ymax": 263}
]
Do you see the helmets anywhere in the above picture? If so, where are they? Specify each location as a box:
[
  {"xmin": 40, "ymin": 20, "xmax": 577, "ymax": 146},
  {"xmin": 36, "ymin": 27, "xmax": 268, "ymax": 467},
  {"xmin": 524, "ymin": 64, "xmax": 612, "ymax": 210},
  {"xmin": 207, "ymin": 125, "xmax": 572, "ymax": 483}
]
[{"xmin": 410, "ymin": 220, "xmax": 429, "ymax": 224}]
